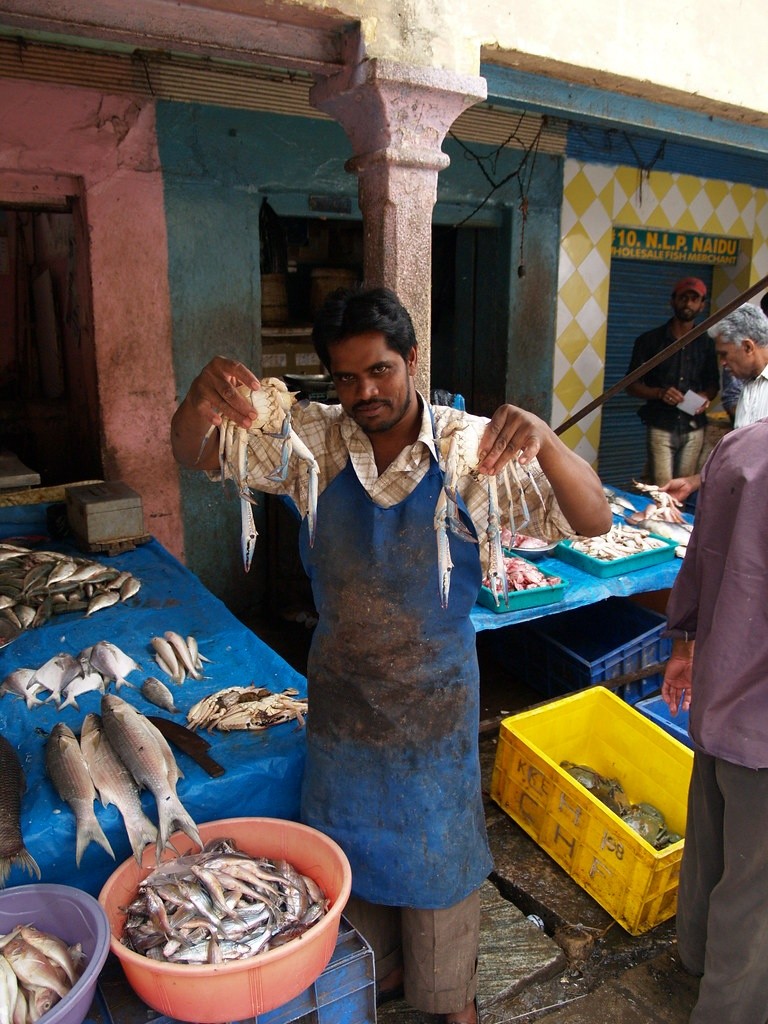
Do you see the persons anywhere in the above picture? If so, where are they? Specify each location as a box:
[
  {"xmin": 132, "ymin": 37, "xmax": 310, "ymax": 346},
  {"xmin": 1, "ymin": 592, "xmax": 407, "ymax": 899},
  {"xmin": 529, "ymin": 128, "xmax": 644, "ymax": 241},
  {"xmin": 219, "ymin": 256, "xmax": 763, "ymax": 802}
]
[
  {"xmin": 168, "ymin": 286, "xmax": 613, "ymax": 1021},
  {"xmin": 627, "ymin": 277, "xmax": 720, "ymax": 504},
  {"xmin": 660, "ymin": 420, "xmax": 767, "ymax": 1024},
  {"xmin": 657, "ymin": 302, "xmax": 768, "ymax": 506}
]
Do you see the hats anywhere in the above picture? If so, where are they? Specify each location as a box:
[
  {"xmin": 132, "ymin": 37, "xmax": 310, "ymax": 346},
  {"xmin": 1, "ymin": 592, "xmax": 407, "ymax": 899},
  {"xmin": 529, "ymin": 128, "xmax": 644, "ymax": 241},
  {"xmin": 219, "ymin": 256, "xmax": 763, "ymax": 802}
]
[{"xmin": 674, "ymin": 277, "xmax": 707, "ymax": 297}]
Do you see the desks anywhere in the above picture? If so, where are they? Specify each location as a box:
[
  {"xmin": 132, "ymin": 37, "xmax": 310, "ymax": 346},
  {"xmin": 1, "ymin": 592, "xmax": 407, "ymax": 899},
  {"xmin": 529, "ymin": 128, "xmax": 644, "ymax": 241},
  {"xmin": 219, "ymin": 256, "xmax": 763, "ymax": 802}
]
[
  {"xmin": 430, "ymin": 481, "xmax": 694, "ymax": 634},
  {"xmin": 1, "ymin": 526, "xmax": 312, "ymax": 908}
]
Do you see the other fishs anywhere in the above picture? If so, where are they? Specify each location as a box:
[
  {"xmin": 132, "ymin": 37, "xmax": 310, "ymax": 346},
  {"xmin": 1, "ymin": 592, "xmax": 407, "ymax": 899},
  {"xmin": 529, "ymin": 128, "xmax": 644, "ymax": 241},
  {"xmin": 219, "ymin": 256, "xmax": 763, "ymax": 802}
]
[
  {"xmin": 0, "ymin": 542, "xmax": 331, "ymax": 1024},
  {"xmin": 603, "ymin": 487, "xmax": 694, "ymax": 559}
]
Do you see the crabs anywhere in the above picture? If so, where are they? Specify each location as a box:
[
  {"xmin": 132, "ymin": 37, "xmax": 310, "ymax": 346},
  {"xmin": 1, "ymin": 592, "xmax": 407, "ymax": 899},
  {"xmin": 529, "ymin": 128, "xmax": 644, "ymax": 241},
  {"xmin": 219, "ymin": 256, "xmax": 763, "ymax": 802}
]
[
  {"xmin": 217, "ymin": 693, "xmax": 309, "ymax": 733},
  {"xmin": 183, "ymin": 682, "xmax": 269, "ymax": 737},
  {"xmin": 194, "ymin": 377, "xmax": 320, "ymax": 572},
  {"xmin": 559, "ymin": 760, "xmax": 683, "ymax": 851},
  {"xmin": 433, "ymin": 418, "xmax": 547, "ymax": 610},
  {"xmin": 633, "ymin": 480, "xmax": 684, "ymax": 514}
]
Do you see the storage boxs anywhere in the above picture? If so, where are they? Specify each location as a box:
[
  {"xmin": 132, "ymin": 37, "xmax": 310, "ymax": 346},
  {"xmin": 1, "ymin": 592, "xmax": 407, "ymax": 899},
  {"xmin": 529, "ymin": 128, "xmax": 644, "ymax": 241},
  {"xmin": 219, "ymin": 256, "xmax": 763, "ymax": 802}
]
[
  {"xmin": 629, "ymin": 692, "xmax": 696, "ymax": 748},
  {"xmin": 58, "ymin": 478, "xmax": 150, "ymax": 539},
  {"xmin": 98, "ymin": 911, "xmax": 380, "ymax": 1023},
  {"xmin": 494, "ymin": 687, "xmax": 698, "ymax": 938},
  {"xmin": 260, "ymin": 344, "xmax": 324, "ymax": 385},
  {"xmin": 541, "ymin": 607, "xmax": 674, "ymax": 708}
]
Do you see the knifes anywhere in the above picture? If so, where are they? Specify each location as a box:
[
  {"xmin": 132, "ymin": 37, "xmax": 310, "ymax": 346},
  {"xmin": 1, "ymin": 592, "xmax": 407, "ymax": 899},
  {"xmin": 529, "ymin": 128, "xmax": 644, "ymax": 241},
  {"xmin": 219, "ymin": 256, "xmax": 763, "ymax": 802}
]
[{"xmin": 146, "ymin": 716, "xmax": 226, "ymax": 778}]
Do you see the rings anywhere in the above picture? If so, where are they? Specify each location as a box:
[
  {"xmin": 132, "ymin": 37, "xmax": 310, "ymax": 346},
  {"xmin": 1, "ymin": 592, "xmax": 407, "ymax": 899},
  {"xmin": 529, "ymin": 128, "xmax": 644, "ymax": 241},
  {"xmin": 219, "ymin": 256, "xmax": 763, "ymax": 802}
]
[{"xmin": 668, "ymin": 397, "xmax": 673, "ymax": 401}]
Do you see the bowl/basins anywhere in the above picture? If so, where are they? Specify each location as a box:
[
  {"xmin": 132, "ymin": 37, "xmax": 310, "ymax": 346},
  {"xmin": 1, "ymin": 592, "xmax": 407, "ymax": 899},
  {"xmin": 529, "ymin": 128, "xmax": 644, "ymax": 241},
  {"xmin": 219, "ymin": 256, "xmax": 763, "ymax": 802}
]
[
  {"xmin": 99, "ymin": 818, "xmax": 352, "ymax": 1023},
  {"xmin": 0, "ymin": 884, "xmax": 111, "ymax": 1024},
  {"xmin": 502, "ymin": 538, "xmax": 559, "ymax": 559}
]
